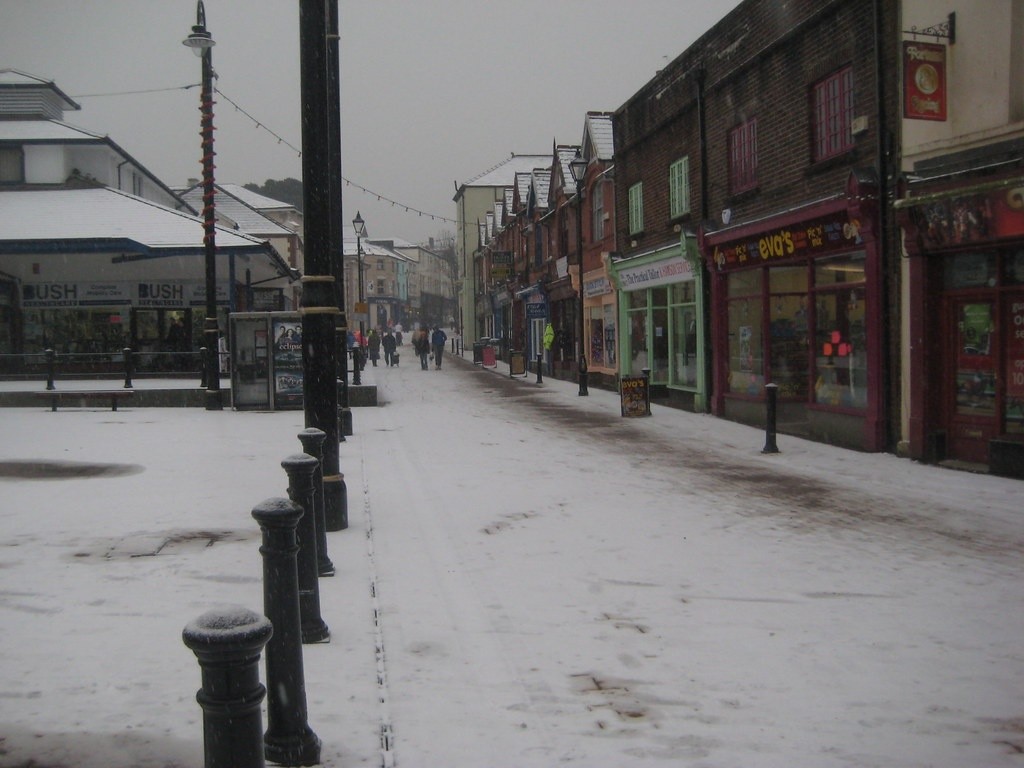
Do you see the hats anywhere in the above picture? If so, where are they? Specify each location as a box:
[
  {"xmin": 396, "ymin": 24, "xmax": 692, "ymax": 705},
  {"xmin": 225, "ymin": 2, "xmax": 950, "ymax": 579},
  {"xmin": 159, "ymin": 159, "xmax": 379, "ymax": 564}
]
[{"xmin": 372, "ymin": 329, "xmax": 377, "ymax": 333}]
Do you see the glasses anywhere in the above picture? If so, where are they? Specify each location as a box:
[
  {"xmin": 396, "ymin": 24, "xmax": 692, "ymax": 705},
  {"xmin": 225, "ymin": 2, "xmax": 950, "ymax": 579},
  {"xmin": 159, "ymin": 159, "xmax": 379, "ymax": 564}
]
[{"xmin": 434, "ymin": 328, "xmax": 436, "ymax": 330}]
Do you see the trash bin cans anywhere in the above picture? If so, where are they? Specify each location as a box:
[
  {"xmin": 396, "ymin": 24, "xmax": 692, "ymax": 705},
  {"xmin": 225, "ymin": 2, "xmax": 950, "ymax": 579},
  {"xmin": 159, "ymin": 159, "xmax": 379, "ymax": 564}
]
[
  {"xmin": 472, "ymin": 342, "xmax": 496, "ymax": 365},
  {"xmin": 480, "ymin": 337, "xmax": 490, "ymax": 346},
  {"xmin": 490, "ymin": 338, "xmax": 502, "ymax": 359},
  {"xmin": 511, "ymin": 350, "xmax": 525, "ymax": 374}
]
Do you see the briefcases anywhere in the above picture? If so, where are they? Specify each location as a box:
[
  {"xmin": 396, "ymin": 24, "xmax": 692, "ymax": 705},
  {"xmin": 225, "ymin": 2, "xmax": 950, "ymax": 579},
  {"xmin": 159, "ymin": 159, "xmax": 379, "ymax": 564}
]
[{"xmin": 394, "ymin": 352, "xmax": 399, "ymax": 364}]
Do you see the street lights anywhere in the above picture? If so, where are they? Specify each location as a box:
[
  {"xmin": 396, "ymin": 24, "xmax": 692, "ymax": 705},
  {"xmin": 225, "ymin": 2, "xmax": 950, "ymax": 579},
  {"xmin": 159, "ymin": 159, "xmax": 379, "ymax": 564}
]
[
  {"xmin": 351, "ymin": 209, "xmax": 366, "ymax": 372},
  {"xmin": 566, "ymin": 146, "xmax": 591, "ymax": 398},
  {"xmin": 181, "ymin": 0, "xmax": 228, "ymax": 413}
]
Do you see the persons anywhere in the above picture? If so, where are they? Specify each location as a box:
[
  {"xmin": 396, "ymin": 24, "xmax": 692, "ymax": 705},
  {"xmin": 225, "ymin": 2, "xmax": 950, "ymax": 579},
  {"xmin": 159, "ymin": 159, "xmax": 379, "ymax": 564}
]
[
  {"xmin": 166, "ymin": 317, "xmax": 185, "ymax": 342},
  {"xmin": 431, "ymin": 325, "xmax": 447, "ymax": 371},
  {"xmin": 415, "ymin": 331, "xmax": 431, "ymax": 371},
  {"xmin": 346, "ymin": 322, "xmax": 403, "ymax": 372},
  {"xmin": 275, "ymin": 325, "xmax": 303, "ymax": 349}
]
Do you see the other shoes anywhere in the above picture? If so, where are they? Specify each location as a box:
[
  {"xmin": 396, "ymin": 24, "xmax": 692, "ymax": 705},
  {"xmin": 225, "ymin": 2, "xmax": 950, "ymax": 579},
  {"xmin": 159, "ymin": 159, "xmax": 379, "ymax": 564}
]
[
  {"xmin": 374, "ymin": 365, "xmax": 378, "ymax": 367},
  {"xmin": 387, "ymin": 364, "xmax": 389, "ymax": 366},
  {"xmin": 422, "ymin": 366, "xmax": 424, "ymax": 369},
  {"xmin": 391, "ymin": 365, "xmax": 393, "ymax": 366},
  {"xmin": 438, "ymin": 366, "xmax": 441, "ymax": 370},
  {"xmin": 434, "ymin": 365, "xmax": 438, "ymax": 369},
  {"xmin": 425, "ymin": 366, "xmax": 428, "ymax": 370}
]
